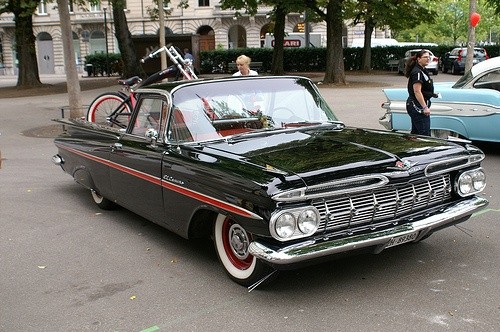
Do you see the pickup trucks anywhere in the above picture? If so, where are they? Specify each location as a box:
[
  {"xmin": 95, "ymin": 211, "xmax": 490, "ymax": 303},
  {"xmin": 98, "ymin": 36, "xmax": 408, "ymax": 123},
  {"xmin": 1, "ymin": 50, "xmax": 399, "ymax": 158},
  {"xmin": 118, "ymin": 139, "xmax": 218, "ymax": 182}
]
[{"xmin": 51, "ymin": 74, "xmax": 491, "ymax": 291}]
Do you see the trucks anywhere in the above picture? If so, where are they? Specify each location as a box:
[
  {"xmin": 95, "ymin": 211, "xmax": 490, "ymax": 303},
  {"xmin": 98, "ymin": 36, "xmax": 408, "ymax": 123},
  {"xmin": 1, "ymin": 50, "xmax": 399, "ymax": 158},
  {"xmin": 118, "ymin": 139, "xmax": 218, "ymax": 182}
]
[{"xmin": 265, "ymin": 33, "xmax": 322, "ymax": 48}]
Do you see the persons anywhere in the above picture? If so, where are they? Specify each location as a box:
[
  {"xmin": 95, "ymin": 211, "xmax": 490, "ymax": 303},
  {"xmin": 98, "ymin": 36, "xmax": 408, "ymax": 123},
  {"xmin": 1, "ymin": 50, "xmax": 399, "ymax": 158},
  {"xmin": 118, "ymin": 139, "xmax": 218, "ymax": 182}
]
[
  {"xmin": 232, "ymin": 55, "xmax": 258, "ymax": 77},
  {"xmin": 406, "ymin": 50, "xmax": 438, "ymax": 137},
  {"xmin": 184, "ymin": 48, "xmax": 195, "ymax": 63}
]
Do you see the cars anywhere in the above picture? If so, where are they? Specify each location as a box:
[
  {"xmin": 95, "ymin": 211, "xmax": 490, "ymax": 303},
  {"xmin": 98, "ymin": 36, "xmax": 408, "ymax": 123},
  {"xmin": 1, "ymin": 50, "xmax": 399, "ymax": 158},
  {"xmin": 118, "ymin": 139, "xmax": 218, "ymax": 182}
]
[
  {"xmin": 397, "ymin": 49, "xmax": 439, "ymax": 75},
  {"xmin": 378, "ymin": 56, "xmax": 500, "ymax": 144},
  {"xmin": 442, "ymin": 47, "xmax": 489, "ymax": 75}
]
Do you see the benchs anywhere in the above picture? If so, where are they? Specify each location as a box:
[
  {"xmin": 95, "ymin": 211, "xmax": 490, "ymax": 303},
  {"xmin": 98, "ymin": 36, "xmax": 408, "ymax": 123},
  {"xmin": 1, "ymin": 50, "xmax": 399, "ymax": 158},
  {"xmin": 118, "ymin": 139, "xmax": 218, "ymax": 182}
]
[
  {"xmin": 228, "ymin": 62, "xmax": 263, "ymax": 73},
  {"xmin": 385, "ymin": 60, "xmax": 400, "ymax": 71}
]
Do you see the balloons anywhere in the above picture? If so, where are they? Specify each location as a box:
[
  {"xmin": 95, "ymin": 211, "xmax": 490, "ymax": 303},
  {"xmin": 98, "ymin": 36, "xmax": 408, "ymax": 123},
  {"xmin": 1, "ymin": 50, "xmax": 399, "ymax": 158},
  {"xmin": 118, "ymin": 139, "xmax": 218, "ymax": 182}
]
[{"xmin": 470, "ymin": 13, "xmax": 480, "ymax": 27}]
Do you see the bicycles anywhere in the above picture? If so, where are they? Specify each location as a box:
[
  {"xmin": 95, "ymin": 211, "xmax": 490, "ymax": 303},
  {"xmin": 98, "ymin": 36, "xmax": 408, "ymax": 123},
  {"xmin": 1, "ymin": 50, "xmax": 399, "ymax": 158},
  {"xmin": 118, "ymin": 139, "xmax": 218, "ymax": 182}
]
[{"xmin": 87, "ymin": 46, "xmax": 203, "ymax": 132}]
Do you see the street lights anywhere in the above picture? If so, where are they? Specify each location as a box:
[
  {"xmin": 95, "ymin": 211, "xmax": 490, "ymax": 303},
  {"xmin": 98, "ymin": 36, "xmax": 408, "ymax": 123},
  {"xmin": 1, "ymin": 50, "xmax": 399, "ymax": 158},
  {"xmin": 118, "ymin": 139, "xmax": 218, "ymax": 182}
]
[{"xmin": 103, "ymin": 7, "xmax": 110, "ymax": 76}]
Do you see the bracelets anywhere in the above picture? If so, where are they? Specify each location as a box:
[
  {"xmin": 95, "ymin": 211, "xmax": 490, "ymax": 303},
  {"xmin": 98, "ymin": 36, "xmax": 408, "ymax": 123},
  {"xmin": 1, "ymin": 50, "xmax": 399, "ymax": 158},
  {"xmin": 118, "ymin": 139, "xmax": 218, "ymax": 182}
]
[{"xmin": 423, "ymin": 106, "xmax": 427, "ymax": 109}]
